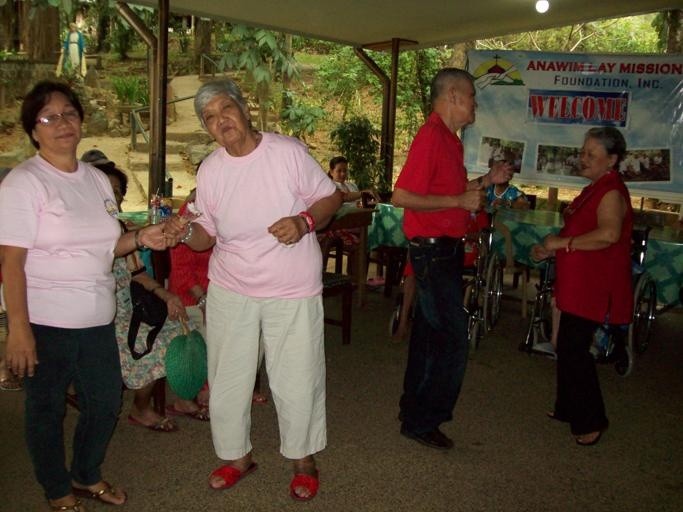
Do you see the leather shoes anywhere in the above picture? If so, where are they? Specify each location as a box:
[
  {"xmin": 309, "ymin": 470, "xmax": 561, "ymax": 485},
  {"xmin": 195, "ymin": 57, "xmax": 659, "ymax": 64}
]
[
  {"xmin": 575, "ymin": 430, "xmax": 602, "ymax": 447},
  {"xmin": 400, "ymin": 422, "xmax": 453, "ymax": 452},
  {"xmin": 546, "ymin": 407, "xmax": 567, "ymax": 422}
]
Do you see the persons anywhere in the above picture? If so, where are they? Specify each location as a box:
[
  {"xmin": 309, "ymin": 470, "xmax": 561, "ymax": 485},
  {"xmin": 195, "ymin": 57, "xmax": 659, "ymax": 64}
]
[
  {"xmin": 325, "ymin": 154, "xmax": 376, "ymax": 207},
  {"xmin": 391, "ymin": 66, "xmax": 516, "ymax": 451},
  {"xmin": 528, "ymin": 126, "xmax": 634, "ymax": 444},
  {"xmin": 0, "ymin": 79, "xmax": 180, "ymax": 512},
  {"xmin": 486, "ymin": 158, "xmax": 530, "ymax": 211},
  {"xmin": 164, "ymin": 78, "xmax": 343, "ymax": 500},
  {"xmin": 59, "ymin": 20, "xmax": 89, "ymax": 79}
]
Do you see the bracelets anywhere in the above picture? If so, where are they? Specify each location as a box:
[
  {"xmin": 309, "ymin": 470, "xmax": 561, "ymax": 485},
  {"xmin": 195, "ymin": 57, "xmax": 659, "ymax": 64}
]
[
  {"xmin": 566, "ymin": 239, "xmax": 573, "ymax": 252},
  {"xmin": 179, "ymin": 223, "xmax": 193, "ymax": 242},
  {"xmin": 298, "ymin": 211, "xmax": 315, "ymax": 233},
  {"xmin": 132, "ymin": 230, "xmax": 149, "ymax": 253},
  {"xmin": 476, "ymin": 176, "xmax": 484, "ymax": 185}
]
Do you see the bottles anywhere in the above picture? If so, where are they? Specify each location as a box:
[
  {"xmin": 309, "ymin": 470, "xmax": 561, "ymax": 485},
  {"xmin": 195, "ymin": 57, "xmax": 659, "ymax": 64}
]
[{"xmin": 148, "ymin": 197, "xmax": 173, "ymax": 225}]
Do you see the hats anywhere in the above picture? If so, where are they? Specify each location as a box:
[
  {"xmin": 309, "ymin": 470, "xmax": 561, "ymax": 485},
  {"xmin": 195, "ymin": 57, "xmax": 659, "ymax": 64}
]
[{"xmin": 79, "ymin": 149, "xmax": 115, "ymax": 169}]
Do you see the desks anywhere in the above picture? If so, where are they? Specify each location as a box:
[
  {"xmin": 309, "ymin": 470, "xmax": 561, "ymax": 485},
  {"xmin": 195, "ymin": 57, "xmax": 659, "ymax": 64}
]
[
  {"xmin": 118, "ymin": 207, "xmax": 175, "ymax": 230},
  {"xmin": 493, "ymin": 205, "xmax": 682, "ymax": 324},
  {"xmin": 365, "ymin": 202, "xmax": 497, "ymax": 299},
  {"xmin": 313, "ymin": 202, "xmax": 378, "ymax": 321}
]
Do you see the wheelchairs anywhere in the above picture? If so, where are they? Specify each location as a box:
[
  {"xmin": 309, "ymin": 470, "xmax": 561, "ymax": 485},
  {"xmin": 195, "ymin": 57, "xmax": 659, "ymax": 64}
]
[
  {"xmin": 387, "ymin": 205, "xmax": 507, "ymax": 352},
  {"xmin": 515, "ymin": 211, "xmax": 664, "ymax": 382}
]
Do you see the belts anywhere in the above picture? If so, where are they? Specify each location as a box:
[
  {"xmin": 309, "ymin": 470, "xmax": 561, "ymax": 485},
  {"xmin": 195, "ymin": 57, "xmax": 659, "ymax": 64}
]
[{"xmin": 409, "ymin": 235, "xmax": 458, "ymax": 247}]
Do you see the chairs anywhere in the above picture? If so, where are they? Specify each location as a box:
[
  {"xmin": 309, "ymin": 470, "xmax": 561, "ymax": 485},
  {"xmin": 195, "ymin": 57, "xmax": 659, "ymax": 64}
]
[
  {"xmin": 318, "ymin": 227, "xmax": 360, "ymax": 346},
  {"xmin": 499, "ymin": 191, "xmax": 539, "ymax": 292}
]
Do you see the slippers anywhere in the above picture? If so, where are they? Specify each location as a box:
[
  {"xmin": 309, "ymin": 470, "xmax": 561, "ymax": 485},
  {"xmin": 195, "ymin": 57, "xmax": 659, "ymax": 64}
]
[
  {"xmin": 532, "ymin": 342, "xmax": 558, "ymax": 359},
  {"xmin": 208, "ymin": 460, "xmax": 257, "ymax": 490},
  {"xmin": 288, "ymin": 465, "xmax": 321, "ymax": 501}
]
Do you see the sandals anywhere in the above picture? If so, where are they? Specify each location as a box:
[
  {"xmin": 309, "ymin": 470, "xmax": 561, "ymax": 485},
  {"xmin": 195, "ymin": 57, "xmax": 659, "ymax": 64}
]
[
  {"xmin": 70, "ymin": 475, "xmax": 128, "ymax": 506},
  {"xmin": 48, "ymin": 490, "xmax": 88, "ymax": 512},
  {"xmin": 166, "ymin": 402, "xmax": 210, "ymax": 423},
  {"xmin": 128, "ymin": 409, "xmax": 176, "ymax": 433}
]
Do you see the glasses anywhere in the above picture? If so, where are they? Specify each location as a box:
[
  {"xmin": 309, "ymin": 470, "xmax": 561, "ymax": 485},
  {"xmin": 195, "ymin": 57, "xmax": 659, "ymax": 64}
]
[{"xmin": 35, "ymin": 110, "xmax": 82, "ymax": 126}]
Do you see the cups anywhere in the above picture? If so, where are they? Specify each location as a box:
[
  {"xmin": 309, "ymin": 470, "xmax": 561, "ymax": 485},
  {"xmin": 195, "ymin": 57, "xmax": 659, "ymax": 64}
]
[{"xmin": 503, "ymin": 192, "xmax": 512, "ymax": 208}]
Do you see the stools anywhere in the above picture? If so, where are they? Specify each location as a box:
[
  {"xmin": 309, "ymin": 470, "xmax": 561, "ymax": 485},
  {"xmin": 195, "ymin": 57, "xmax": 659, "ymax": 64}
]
[
  {"xmin": 318, "ymin": 233, "xmax": 344, "ymax": 276},
  {"xmin": 152, "ymin": 376, "xmax": 165, "ymax": 418}
]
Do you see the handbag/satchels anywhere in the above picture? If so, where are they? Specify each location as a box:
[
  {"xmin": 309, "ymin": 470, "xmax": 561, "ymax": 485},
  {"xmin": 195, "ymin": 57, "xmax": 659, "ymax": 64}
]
[{"xmin": 127, "ymin": 280, "xmax": 168, "ymax": 359}]
